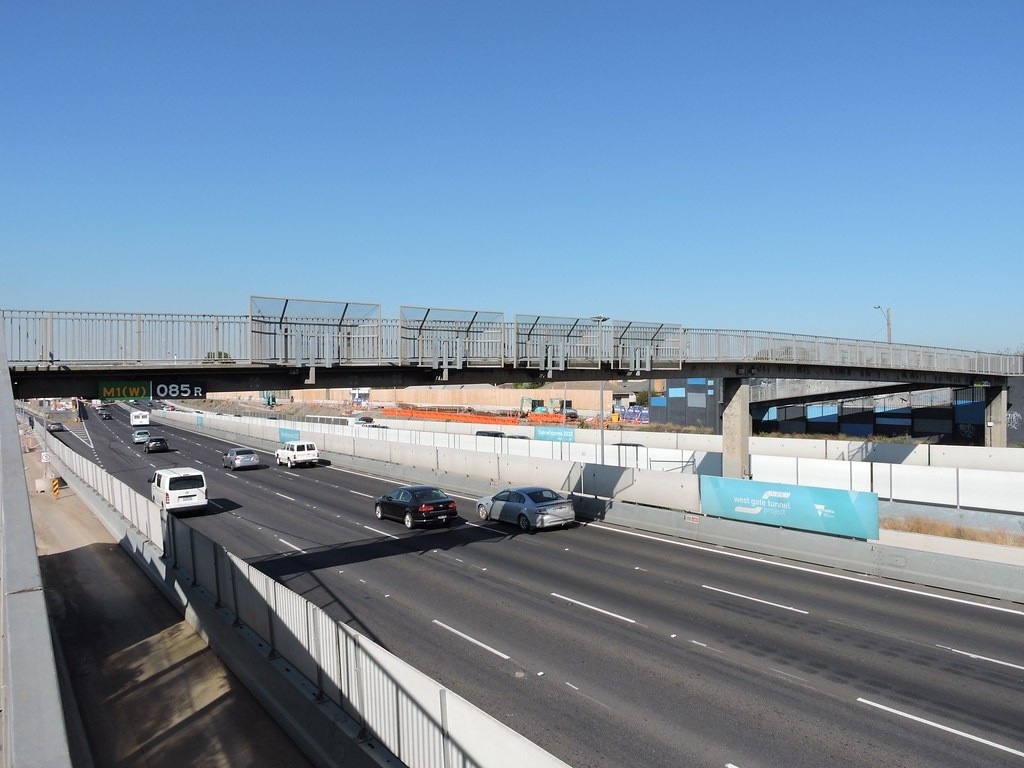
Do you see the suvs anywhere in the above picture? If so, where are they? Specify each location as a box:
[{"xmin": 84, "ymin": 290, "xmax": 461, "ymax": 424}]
[{"xmin": 274, "ymin": 442, "xmax": 320, "ymax": 468}]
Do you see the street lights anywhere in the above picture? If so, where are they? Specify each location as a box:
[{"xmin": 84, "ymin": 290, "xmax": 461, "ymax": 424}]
[
  {"xmin": 591, "ymin": 315, "xmax": 610, "ymax": 465},
  {"xmin": 168, "ymin": 352, "xmax": 177, "ymax": 365},
  {"xmin": 875, "ymin": 305, "xmax": 892, "ymax": 344}
]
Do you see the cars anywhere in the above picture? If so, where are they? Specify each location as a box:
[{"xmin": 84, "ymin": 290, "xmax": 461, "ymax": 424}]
[
  {"xmin": 132, "ymin": 430, "xmax": 151, "ymax": 445},
  {"xmin": 48, "ymin": 423, "xmax": 63, "ymax": 431},
  {"xmin": 374, "ymin": 486, "xmax": 458, "ymax": 529},
  {"xmin": 148, "ymin": 399, "xmax": 241, "ymax": 417},
  {"xmin": 475, "ymin": 486, "xmax": 576, "ymax": 531},
  {"xmin": 222, "ymin": 448, "xmax": 259, "ymax": 471},
  {"xmin": 96, "ymin": 402, "xmax": 112, "ymax": 419},
  {"xmin": 133, "ymin": 400, "xmax": 138, "ymax": 405},
  {"xmin": 144, "ymin": 437, "xmax": 169, "ymax": 454}
]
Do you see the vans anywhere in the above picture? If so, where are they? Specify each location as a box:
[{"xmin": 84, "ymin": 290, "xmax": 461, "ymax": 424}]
[{"xmin": 147, "ymin": 466, "xmax": 210, "ymax": 513}]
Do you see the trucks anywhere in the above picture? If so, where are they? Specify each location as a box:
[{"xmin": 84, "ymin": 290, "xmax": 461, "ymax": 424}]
[
  {"xmin": 129, "ymin": 411, "xmax": 150, "ymax": 426},
  {"xmin": 92, "ymin": 399, "xmax": 101, "ymax": 408}
]
